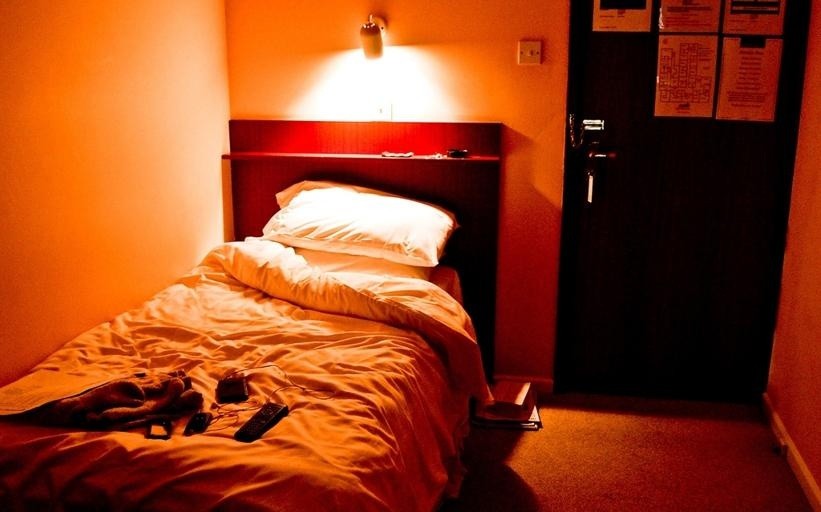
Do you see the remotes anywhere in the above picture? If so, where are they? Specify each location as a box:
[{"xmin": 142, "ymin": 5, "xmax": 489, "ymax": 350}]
[{"xmin": 233, "ymin": 402, "xmax": 288, "ymax": 443}]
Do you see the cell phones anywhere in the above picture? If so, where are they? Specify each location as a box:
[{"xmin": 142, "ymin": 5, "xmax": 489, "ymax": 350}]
[{"xmin": 182, "ymin": 412, "xmax": 212, "ymax": 437}]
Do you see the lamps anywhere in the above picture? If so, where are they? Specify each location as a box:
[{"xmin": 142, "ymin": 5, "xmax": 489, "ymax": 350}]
[{"xmin": 361, "ymin": 11, "xmax": 388, "ymax": 61}]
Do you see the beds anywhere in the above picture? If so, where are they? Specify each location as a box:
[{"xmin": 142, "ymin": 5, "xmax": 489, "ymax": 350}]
[{"xmin": 2, "ymin": 256, "xmax": 485, "ymax": 512}]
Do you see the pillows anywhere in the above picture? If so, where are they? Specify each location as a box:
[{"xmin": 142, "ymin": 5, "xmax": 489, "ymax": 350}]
[{"xmin": 254, "ymin": 179, "xmax": 459, "ymax": 285}]
